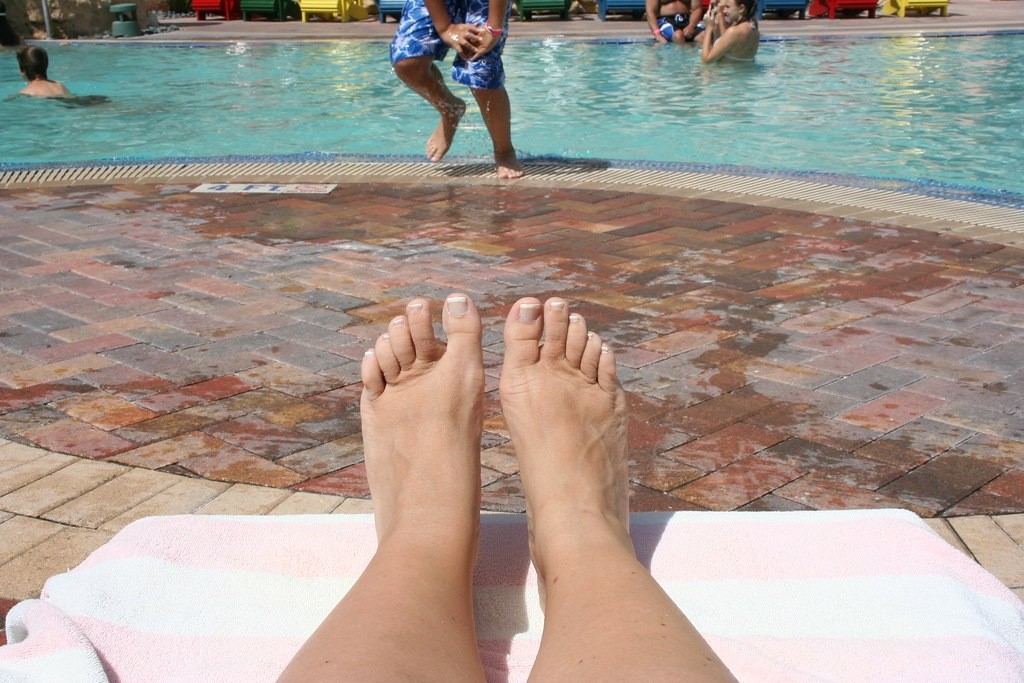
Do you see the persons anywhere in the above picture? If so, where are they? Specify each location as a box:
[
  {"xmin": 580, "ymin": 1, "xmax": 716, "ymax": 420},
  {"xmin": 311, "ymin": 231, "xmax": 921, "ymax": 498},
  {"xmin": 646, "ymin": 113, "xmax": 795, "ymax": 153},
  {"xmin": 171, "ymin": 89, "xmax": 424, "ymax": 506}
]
[
  {"xmin": 389, "ymin": 0, "xmax": 526, "ymax": 178},
  {"xmin": 270, "ymin": 292, "xmax": 738, "ymax": 683},
  {"xmin": 652, "ymin": 28, "xmax": 661, "ymax": 35},
  {"xmin": 16, "ymin": 45, "xmax": 72, "ymax": 97},
  {"xmin": 645, "ymin": 0, "xmax": 761, "ymax": 68}
]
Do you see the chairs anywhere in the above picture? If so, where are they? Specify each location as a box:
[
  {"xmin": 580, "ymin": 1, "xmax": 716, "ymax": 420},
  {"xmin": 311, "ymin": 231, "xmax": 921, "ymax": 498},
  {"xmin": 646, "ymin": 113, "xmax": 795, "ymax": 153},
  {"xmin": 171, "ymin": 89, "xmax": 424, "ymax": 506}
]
[{"xmin": 0, "ymin": 508, "xmax": 1024, "ymax": 683}]
